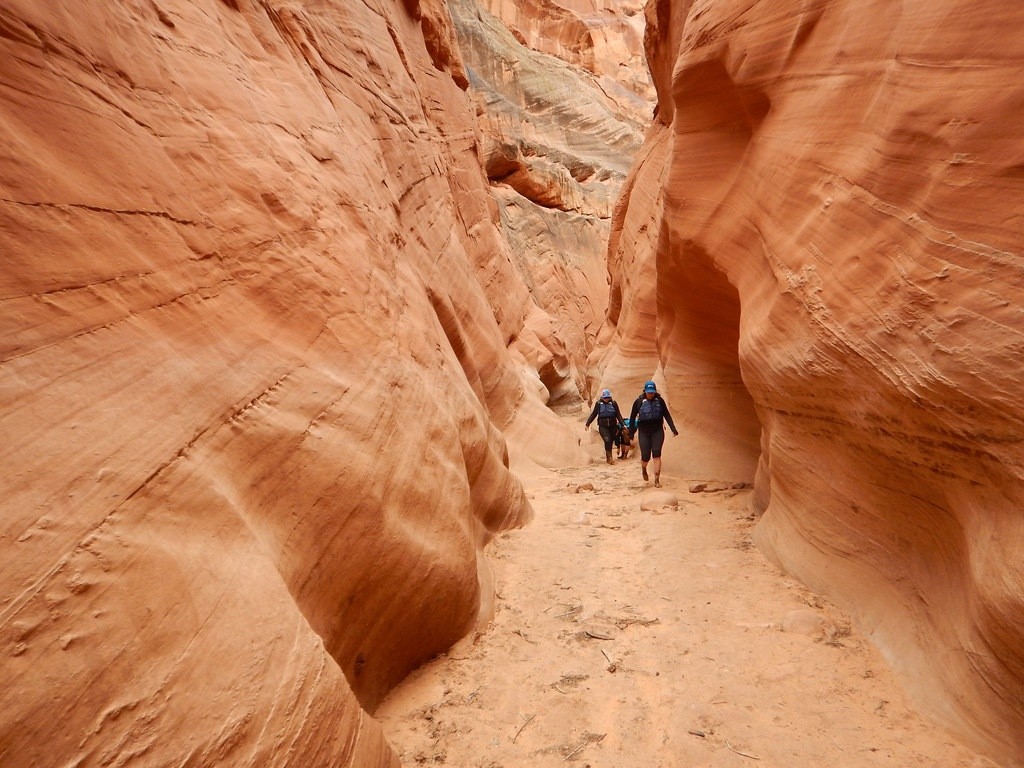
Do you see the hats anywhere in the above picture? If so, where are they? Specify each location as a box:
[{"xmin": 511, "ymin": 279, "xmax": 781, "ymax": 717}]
[
  {"xmin": 601, "ymin": 390, "xmax": 612, "ymax": 398},
  {"xmin": 643, "ymin": 381, "xmax": 657, "ymax": 394}
]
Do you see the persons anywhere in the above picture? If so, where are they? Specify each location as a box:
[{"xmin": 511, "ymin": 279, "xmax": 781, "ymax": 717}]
[
  {"xmin": 628, "ymin": 379, "xmax": 679, "ymax": 489},
  {"xmin": 585, "ymin": 388, "xmax": 627, "ymax": 465},
  {"xmin": 615, "ymin": 417, "xmax": 636, "ymax": 460}
]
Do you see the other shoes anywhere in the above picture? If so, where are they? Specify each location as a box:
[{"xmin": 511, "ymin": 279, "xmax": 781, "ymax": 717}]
[
  {"xmin": 655, "ymin": 482, "xmax": 662, "ymax": 487},
  {"xmin": 607, "ymin": 456, "xmax": 615, "ymax": 464},
  {"xmin": 622, "ymin": 454, "xmax": 628, "ymax": 459},
  {"xmin": 642, "ymin": 469, "xmax": 649, "ymax": 481},
  {"xmin": 617, "ymin": 453, "xmax": 624, "ymax": 459}
]
